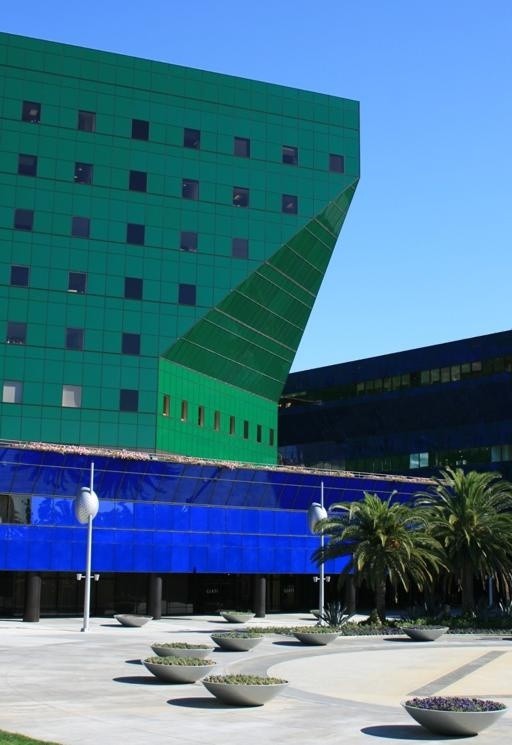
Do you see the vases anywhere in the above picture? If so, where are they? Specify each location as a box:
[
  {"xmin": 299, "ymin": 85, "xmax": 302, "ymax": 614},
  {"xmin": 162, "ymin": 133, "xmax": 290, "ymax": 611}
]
[
  {"xmin": 114, "ymin": 614, "xmax": 154, "ymax": 627},
  {"xmin": 399, "ymin": 700, "xmax": 509, "ymax": 735}
]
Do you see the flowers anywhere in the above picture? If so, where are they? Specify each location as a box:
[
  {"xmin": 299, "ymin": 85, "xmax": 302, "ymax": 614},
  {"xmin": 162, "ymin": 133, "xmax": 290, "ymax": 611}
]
[{"xmin": 408, "ymin": 697, "xmax": 505, "ymax": 713}]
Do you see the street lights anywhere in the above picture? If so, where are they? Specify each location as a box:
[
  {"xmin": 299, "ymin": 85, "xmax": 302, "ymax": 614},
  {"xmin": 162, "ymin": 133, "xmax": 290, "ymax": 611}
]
[
  {"xmin": 67, "ymin": 459, "xmax": 104, "ymax": 633},
  {"xmin": 307, "ymin": 480, "xmax": 332, "ymax": 628}
]
